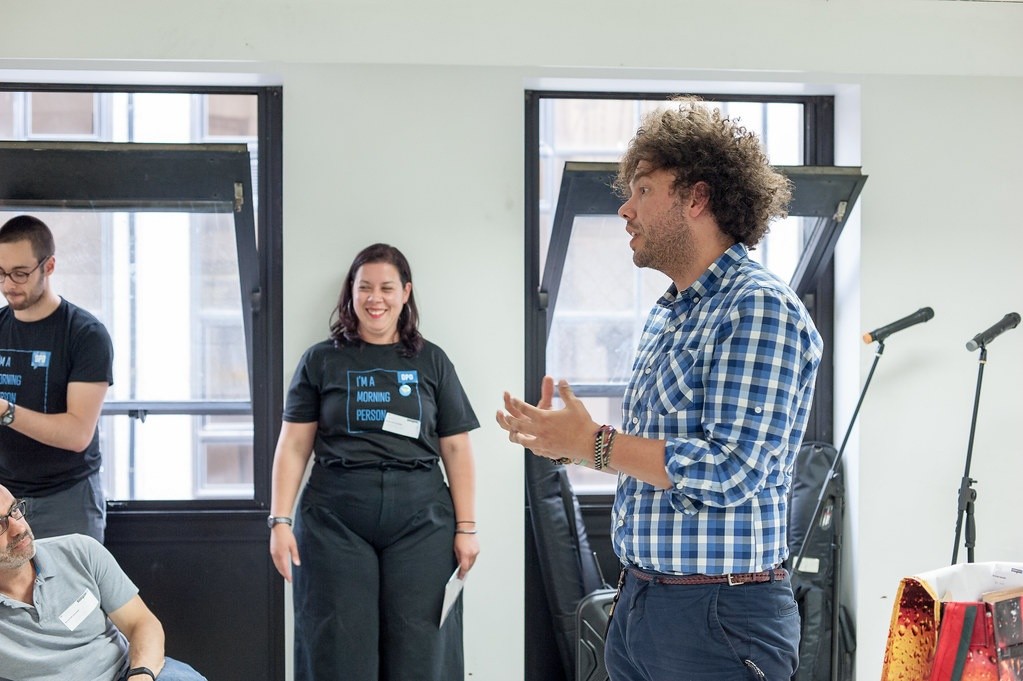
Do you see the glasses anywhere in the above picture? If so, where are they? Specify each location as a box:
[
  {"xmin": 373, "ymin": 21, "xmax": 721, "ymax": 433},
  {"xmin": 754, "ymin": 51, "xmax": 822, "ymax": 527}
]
[
  {"xmin": 0, "ymin": 499, "xmax": 27, "ymax": 536},
  {"xmin": 0, "ymin": 254, "xmax": 50, "ymax": 283}
]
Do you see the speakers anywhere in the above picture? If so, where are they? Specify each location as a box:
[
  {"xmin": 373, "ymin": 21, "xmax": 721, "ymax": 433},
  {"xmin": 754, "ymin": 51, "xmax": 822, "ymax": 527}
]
[{"xmin": 574, "ymin": 588, "xmax": 621, "ymax": 681}]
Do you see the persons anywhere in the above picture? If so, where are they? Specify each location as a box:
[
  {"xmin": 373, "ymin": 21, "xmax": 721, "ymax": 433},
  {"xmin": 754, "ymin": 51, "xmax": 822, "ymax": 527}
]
[
  {"xmin": 267, "ymin": 244, "xmax": 481, "ymax": 681},
  {"xmin": 0, "ymin": 485, "xmax": 208, "ymax": 681},
  {"xmin": 496, "ymin": 103, "xmax": 824, "ymax": 681},
  {"xmin": 0, "ymin": 214, "xmax": 114, "ymax": 545}
]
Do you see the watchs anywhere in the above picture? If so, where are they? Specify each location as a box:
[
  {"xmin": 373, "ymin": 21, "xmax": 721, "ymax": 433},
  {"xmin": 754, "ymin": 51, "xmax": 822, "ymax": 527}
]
[
  {"xmin": 0, "ymin": 402, "xmax": 15, "ymax": 426},
  {"xmin": 126, "ymin": 667, "xmax": 155, "ymax": 681},
  {"xmin": 267, "ymin": 516, "xmax": 292, "ymax": 528}
]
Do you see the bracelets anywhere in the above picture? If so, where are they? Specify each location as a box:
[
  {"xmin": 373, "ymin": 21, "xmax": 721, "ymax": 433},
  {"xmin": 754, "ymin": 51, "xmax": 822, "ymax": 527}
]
[
  {"xmin": 593, "ymin": 424, "xmax": 618, "ymax": 470},
  {"xmin": 456, "ymin": 531, "xmax": 476, "ymax": 534},
  {"xmin": 551, "ymin": 457, "xmax": 587, "ymax": 467},
  {"xmin": 457, "ymin": 521, "xmax": 476, "ymax": 523}
]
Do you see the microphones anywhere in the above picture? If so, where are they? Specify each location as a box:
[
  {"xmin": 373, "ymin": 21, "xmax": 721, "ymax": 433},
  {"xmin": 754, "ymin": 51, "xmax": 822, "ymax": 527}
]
[
  {"xmin": 863, "ymin": 307, "xmax": 935, "ymax": 344},
  {"xmin": 966, "ymin": 312, "xmax": 1021, "ymax": 352}
]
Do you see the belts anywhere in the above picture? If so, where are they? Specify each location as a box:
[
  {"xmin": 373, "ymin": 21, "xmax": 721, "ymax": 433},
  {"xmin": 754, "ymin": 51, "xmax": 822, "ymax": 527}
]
[{"xmin": 632, "ymin": 566, "xmax": 789, "ymax": 585}]
